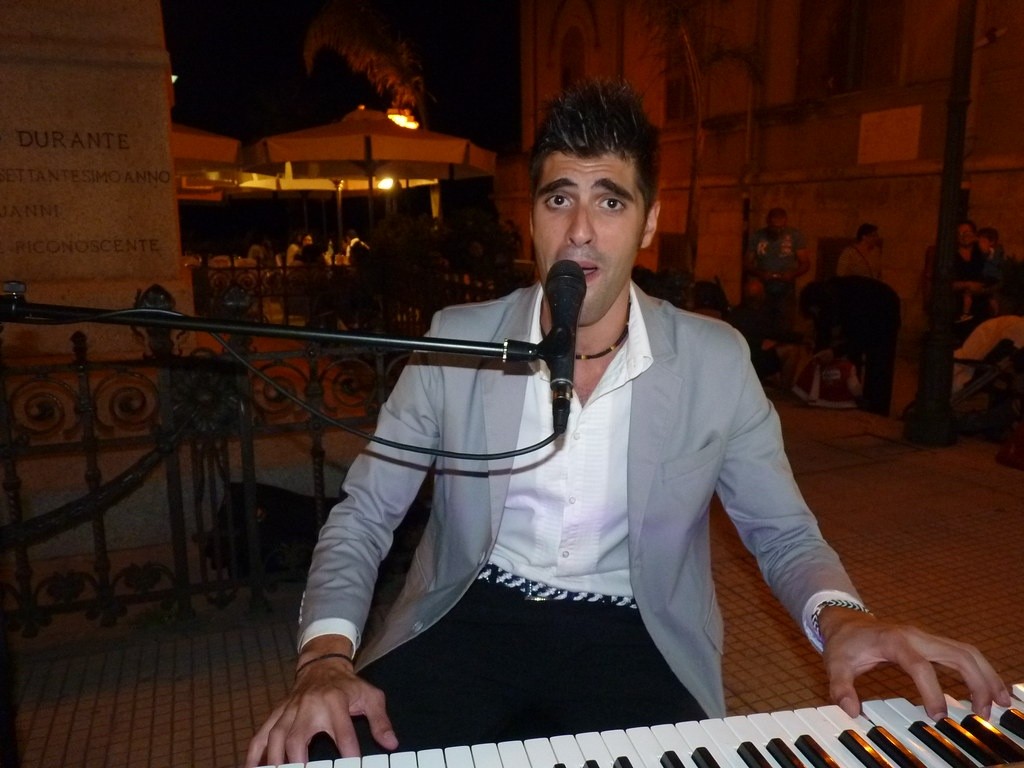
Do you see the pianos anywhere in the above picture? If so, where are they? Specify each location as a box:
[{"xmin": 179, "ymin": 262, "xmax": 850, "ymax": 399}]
[{"xmin": 250, "ymin": 681, "xmax": 1023, "ymax": 768}]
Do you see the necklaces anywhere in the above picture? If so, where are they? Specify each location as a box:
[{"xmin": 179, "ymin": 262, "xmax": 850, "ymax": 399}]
[{"xmin": 538, "ymin": 297, "xmax": 631, "ymax": 361}]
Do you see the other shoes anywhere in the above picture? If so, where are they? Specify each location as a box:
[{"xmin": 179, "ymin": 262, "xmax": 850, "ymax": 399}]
[{"xmin": 954, "ymin": 313, "xmax": 974, "ymax": 323}]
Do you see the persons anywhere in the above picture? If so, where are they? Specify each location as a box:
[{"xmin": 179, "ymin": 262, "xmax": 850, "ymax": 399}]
[
  {"xmin": 245, "ymin": 81, "xmax": 1012, "ymax": 768},
  {"xmin": 250, "ymin": 229, "xmax": 381, "ymax": 332},
  {"xmin": 749, "ymin": 205, "xmax": 810, "ymax": 310},
  {"xmin": 944, "ymin": 216, "xmax": 1005, "ymax": 341},
  {"xmin": 837, "ymin": 222, "xmax": 879, "ymax": 279},
  {"xmin": 798, "ymin": 274, "xmax": 899, "ymax": 417}
]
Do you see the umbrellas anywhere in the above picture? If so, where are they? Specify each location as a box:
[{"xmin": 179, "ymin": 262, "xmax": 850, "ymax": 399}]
[{"xmin": 235, "ymin": 106, "xmax": 497, "ymax": 230}]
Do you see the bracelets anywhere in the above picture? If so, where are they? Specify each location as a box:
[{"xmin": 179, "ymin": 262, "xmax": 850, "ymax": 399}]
[
  {"xmin": 294, "ymin": 653, "xmax": 355, "ymax": 677},
  {"xmin": 810, "ymin": 600, "xmax": 872, "ymax": 643}
]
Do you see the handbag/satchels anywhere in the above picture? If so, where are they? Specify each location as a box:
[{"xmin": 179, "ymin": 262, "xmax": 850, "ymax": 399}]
[{"xmin": 790, "ymin": 354, "xmax": 856, "ymax": 408}]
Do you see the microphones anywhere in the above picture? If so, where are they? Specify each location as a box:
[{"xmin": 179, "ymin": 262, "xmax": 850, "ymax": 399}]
[{"xmin": 545, "ymin": 259, "xmax": 588, "ymax": 433}]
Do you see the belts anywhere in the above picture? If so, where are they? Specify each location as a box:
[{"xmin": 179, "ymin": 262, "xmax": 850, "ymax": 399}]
[{"xmin": 478, "ymin": 562, "xmax": 638, "ymax": 610}]
